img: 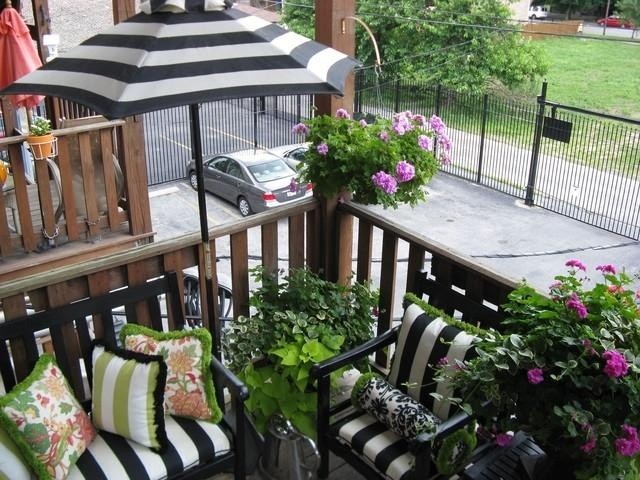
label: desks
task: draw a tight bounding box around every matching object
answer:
[457,429,543,480]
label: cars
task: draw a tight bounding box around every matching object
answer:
[185,143,312,217]
[597,16,631,29]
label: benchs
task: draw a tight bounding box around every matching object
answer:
[0,270,250,480]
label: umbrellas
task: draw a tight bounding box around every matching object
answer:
[0,0,370,358]
[1,0,52,135]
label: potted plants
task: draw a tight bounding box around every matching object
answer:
[27,116,54,158]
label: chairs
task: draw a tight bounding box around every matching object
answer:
[307,323,493,480]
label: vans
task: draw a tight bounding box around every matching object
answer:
[528,6,547,19]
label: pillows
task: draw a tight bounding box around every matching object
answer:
[388,294,504,433]
[0,352,98,480]
[0,424,35,479]
[119,321,223,425]
[358,378,443,446]
[89,336,170,454]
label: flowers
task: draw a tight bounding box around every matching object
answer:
[399,258,640,480]
[282,111,452,210]
